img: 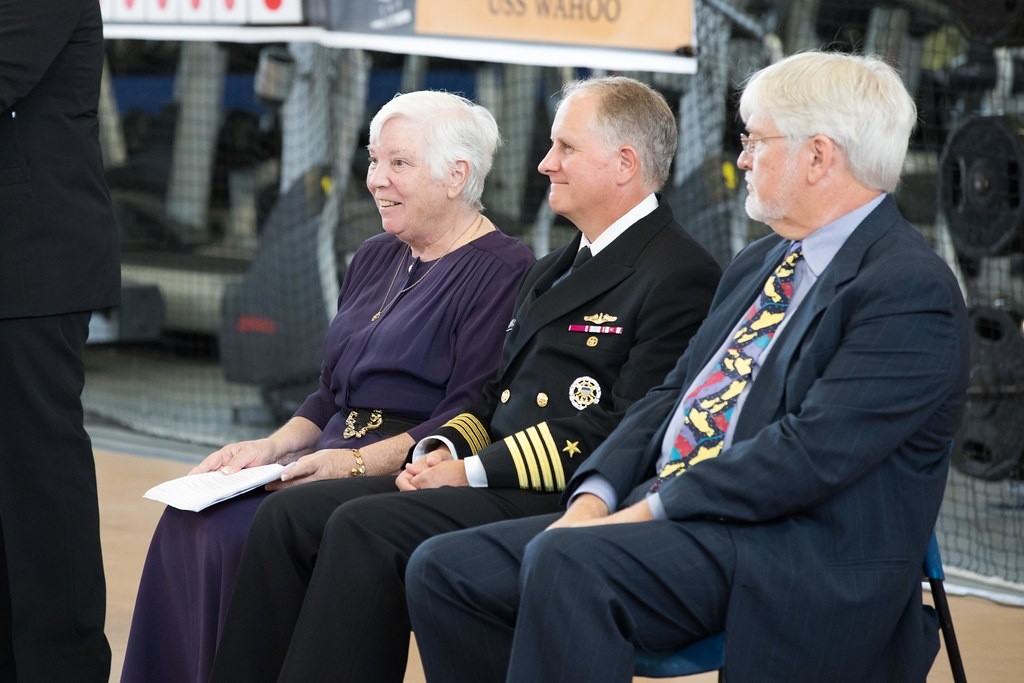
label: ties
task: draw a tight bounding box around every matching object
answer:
[571,245,592,274]
[644,245,804,493]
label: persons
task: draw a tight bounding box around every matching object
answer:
[0,0,122,683]
[119,90,537,683]
[405,49,971,683]
[205,77,724,683]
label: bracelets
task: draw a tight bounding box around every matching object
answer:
[347,449,366,478]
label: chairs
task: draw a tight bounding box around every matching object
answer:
[633,533,967,683]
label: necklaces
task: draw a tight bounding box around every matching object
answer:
[371,213,483,321]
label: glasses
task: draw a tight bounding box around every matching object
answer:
[739,133,788,149]
